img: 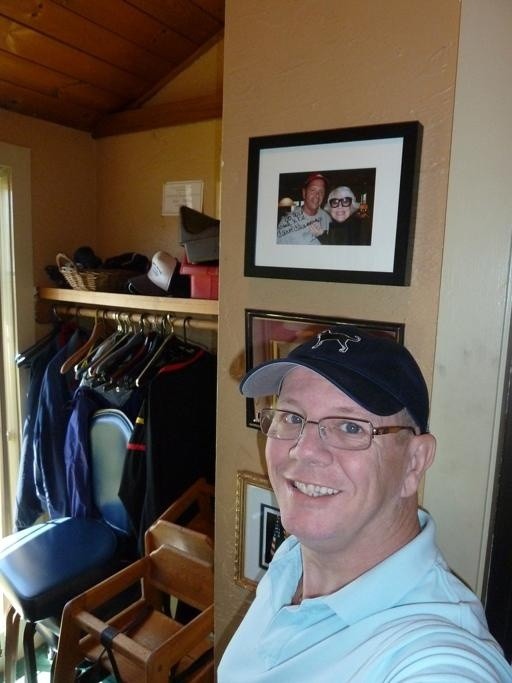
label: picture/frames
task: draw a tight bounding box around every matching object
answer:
[243,307,406,430]
[233,467,293,594]
[243,118,424,287]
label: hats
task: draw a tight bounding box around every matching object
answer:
[278,197,297,208]
[238,324,429,435]
[304,173,327,186]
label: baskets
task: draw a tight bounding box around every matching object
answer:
[50,251,139,293]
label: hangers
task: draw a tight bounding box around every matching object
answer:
[14,305,199,391]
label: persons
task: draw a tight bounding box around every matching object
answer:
[216,325,512,683]
[277,171,371,245]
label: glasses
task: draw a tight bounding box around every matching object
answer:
[329,197,352,208]
[253,409,419,451]
